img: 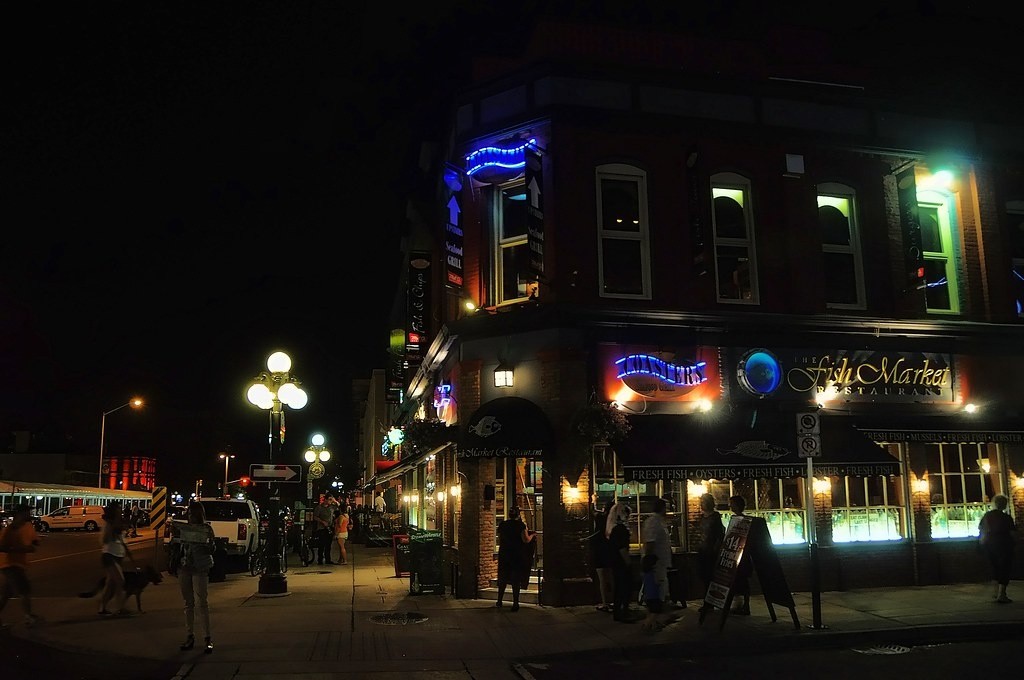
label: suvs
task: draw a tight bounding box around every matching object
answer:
[41,506,106,532]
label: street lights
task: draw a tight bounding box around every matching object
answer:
[303,431,332,548]
[220,454,236,497]
[245,352,308,597]
[99,401,143,489]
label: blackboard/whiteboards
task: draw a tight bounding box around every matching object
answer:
[409,531,446,595]
[393,534,410,572]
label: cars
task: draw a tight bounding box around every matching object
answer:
[0,509,13,528]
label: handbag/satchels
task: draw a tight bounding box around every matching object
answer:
[976,544,992,561]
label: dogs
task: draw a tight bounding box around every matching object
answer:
[80,570,167,615]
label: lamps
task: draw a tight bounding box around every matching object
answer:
[494,364,515,387]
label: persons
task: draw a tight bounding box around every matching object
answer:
[313,493,335,565]
[97,501,137,615]
[589,493,623,621]
[693,493,750,615]
[640,499,672,631]
[496,507,537,612]
[351,504,371,525]
[978,494,1016,604]
[375,491,386,516]
[179,502,216,653]
[124,506,137,536]
[0,504,44,624]
[933,494,943,504]
[334,504,349,565]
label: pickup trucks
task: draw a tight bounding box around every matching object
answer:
[163,498,259,572]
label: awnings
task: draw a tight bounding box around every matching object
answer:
[456,396,554,462]
[823,401,1024,445]
[591,401,902,485]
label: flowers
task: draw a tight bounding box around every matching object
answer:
[401,417,448,456]
[567,401,632,462]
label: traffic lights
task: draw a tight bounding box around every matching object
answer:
[242,479,247,483]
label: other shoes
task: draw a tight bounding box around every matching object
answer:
[992,592,1012,604]
[732,608,750,616]
[325,560,337,565]
[117,609,134,616]
[337,559,347,565]
[496,601,501,606]
[697,606,714,613]
[597,603,609,613]
[511,603,519,609]
[204,641,214,654]
[26,616,38,629]
[180,640,193,651]
[98,609,112,617]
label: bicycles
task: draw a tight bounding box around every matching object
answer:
[250,535,288,577]
[299,536,316,566]
